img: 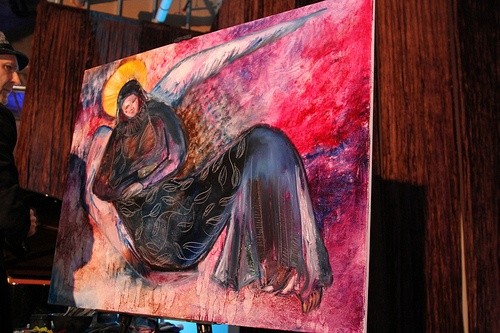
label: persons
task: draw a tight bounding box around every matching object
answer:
[0,30,37,306]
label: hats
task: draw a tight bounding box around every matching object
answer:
[0,30,29,71]
